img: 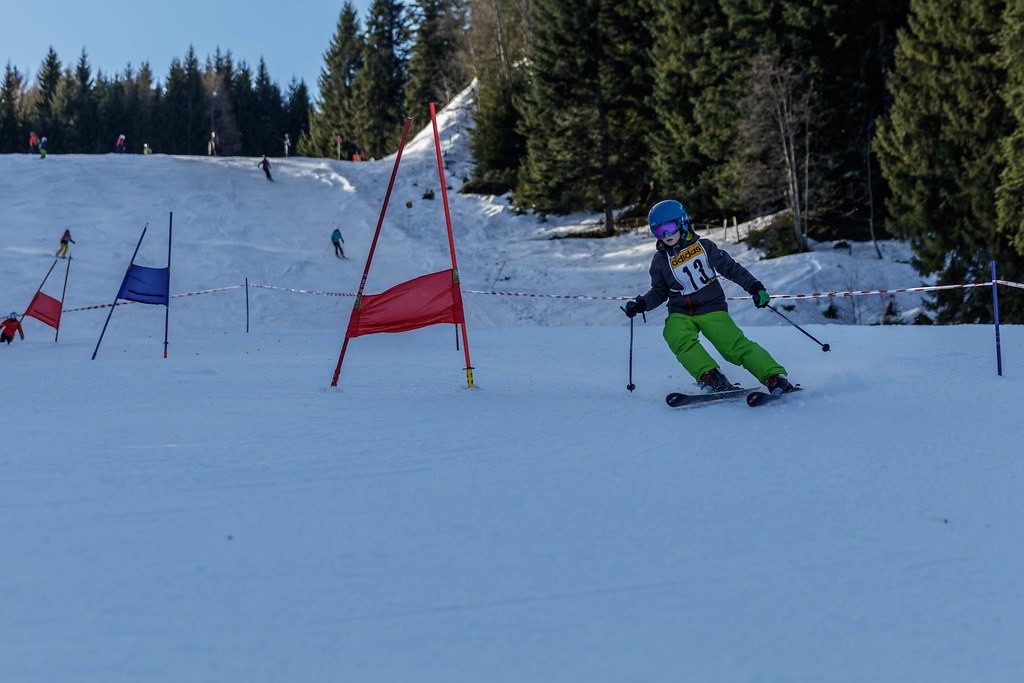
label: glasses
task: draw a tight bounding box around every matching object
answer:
[649,219,682,240]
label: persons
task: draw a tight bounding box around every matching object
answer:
[258,155,273,181]
[207,129,219,155]
[116,134,126,154]
[0,311,24,345]
[335,134,342,160]
[347,147,375,161]
[283,133,291,156]
[332,226,344,258]
[624,200,795,394]
[28,130,47,159]
[143,142,152,155]
[55,229,77,257]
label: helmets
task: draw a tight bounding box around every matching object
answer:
[648,199,688,240]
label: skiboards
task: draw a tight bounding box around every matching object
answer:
[664,382,803,408]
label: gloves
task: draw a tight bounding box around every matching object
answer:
[746,282,770,308]
[625,301,644,318]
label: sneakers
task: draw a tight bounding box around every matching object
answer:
[691,369,744,393]
[762,374,795,400]
